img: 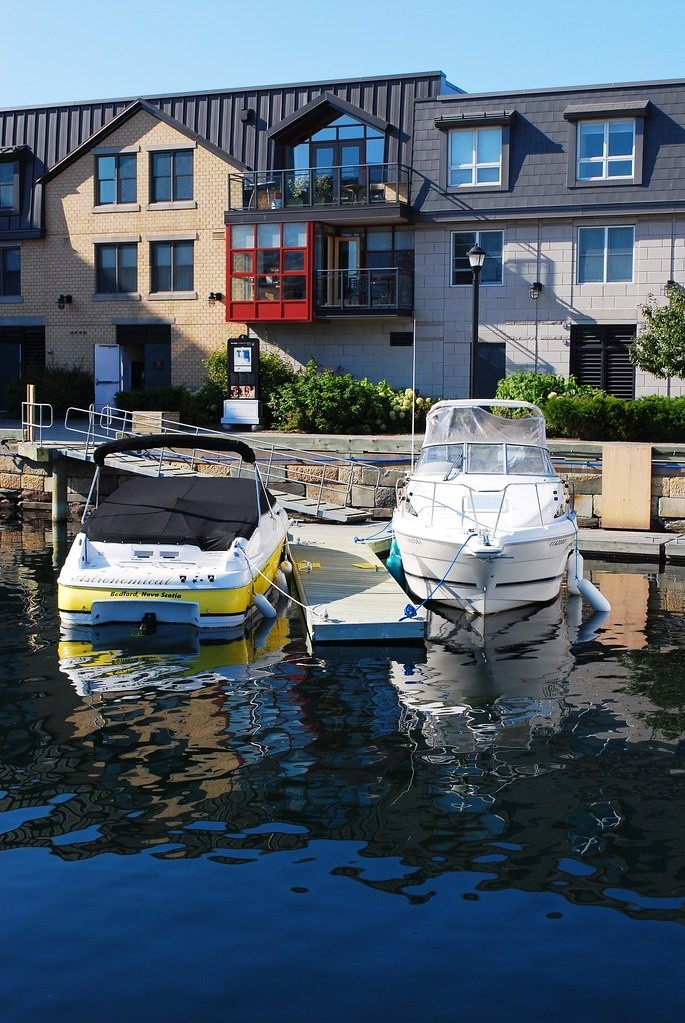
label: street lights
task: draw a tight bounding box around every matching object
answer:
[467,241,489,403]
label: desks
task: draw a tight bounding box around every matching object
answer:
[345,184,366,206]
[248,282,300,301]
[244,181,277,210]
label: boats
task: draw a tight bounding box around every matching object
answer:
[54,435,290,648]
[56,601,297,698]
[391,589,576,711]
[387,318,610,617]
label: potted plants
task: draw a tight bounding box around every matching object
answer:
[116,384,194,433]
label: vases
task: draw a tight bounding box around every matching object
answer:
[285,194,331,207]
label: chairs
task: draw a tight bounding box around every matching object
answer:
[349,275,395,305]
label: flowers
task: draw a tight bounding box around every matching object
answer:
[288,173,332,199]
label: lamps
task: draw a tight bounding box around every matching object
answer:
[55,294,73,311]
[209,292,222,308]
[530,282,542,299]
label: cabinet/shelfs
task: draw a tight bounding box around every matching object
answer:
[370,182,409,203]
